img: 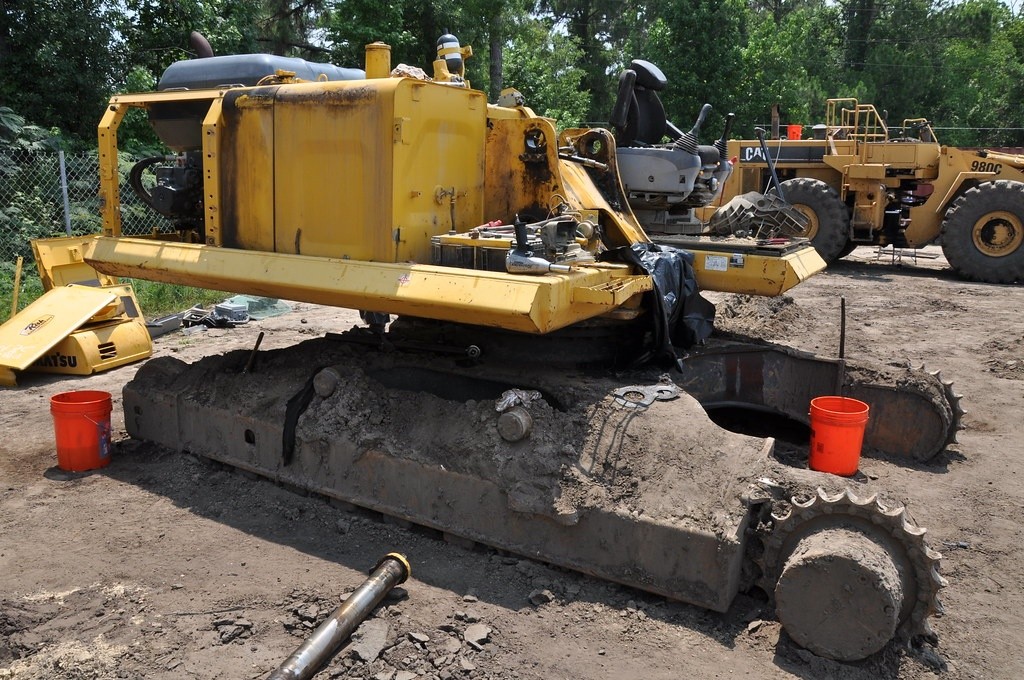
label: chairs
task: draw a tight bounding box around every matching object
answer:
[880,110,917,142]
[609,58,721,166]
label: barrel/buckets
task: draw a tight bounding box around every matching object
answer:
[50,390,113,471]
[808,396,869,476]
[787,125,802,140]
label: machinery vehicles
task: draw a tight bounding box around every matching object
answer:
[696,98,1024,285]
[86,38,970,680]
